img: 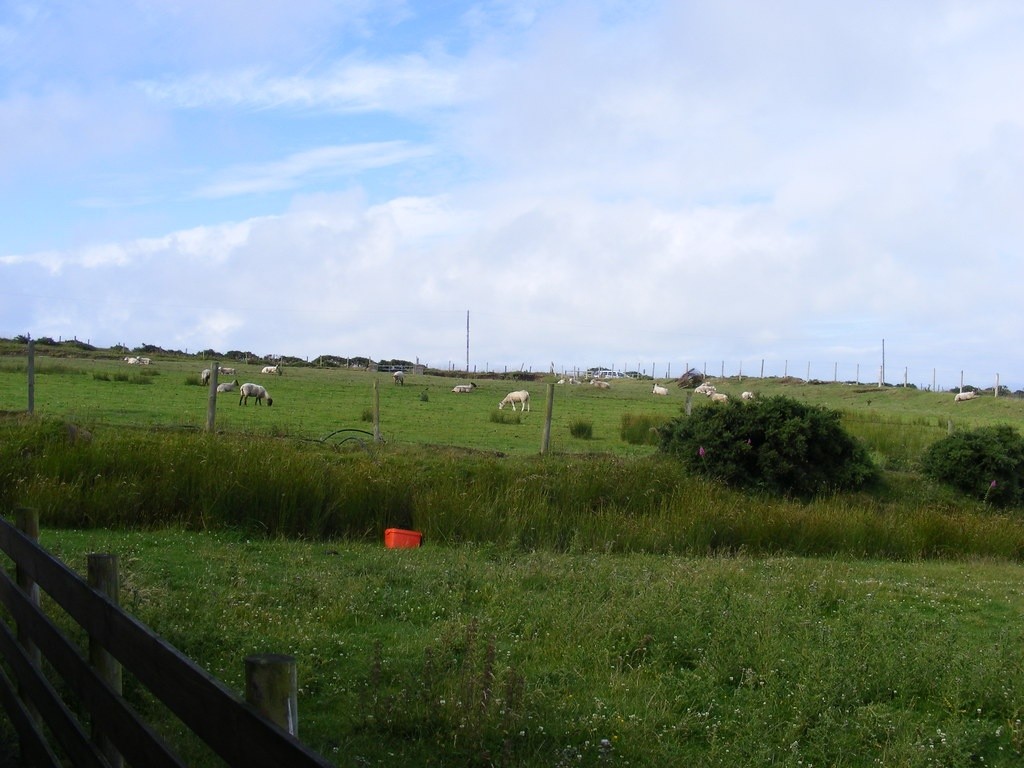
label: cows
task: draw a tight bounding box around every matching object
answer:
[742,391,756,399]
[499,390,531,412]
[124,356,153,366]
[558,378,581,385]
[392,371,404,385]
[239,383,273,406]
[261,365,278,373]
[652,382,668,396]
[216,379,239,392]
[710,390,728,403]
[590,379,609,389]
[202,368,211,386]
[218,367,236,375]
[451,382,477,393]
[955,390,976,401]
[694,381,716,396]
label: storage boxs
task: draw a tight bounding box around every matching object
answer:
[384,528,422,549]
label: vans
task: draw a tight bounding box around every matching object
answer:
[586,371,627,379]
